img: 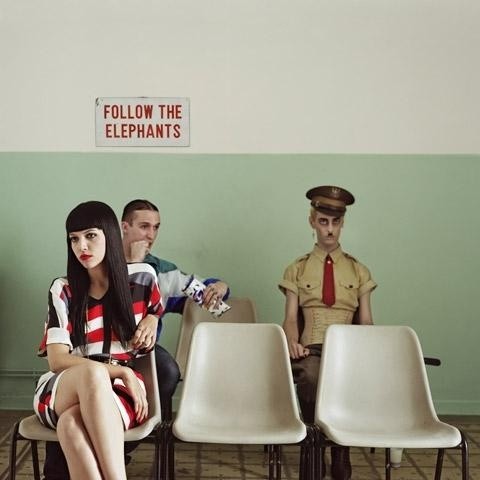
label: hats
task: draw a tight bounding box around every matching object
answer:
[306,187,355,217]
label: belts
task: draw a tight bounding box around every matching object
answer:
[83,355,134,369]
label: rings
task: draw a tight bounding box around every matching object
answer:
[213,295,218,301]
[138,327,144,334]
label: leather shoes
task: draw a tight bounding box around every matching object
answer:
[330,446,351,480]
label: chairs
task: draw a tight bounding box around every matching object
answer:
[167,322,313,480]
[170,296,259,381]
[9,343,166,479]
[314,322,467,478]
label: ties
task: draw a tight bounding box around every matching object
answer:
[320,253,335,308]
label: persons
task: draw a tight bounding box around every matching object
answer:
[44,200,229,479]
[33,200,165,479]
[277,186,379,478]
